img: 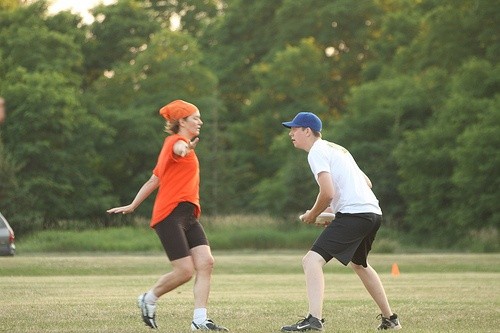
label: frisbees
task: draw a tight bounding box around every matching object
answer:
[300,211,335,225]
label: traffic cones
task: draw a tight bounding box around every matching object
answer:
[392,262,400,276]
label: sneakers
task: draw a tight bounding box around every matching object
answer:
[376,313,402,331]
[136,292,158,329]
[192,319,228,332]
[280,313,325,331]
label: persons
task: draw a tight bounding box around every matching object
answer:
[106,99,229,332]
[280,112,402,331]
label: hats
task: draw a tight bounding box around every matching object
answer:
[282,112,322,133]
[159,99,198,122]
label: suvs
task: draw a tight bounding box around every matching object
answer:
[1,213,17,257]
